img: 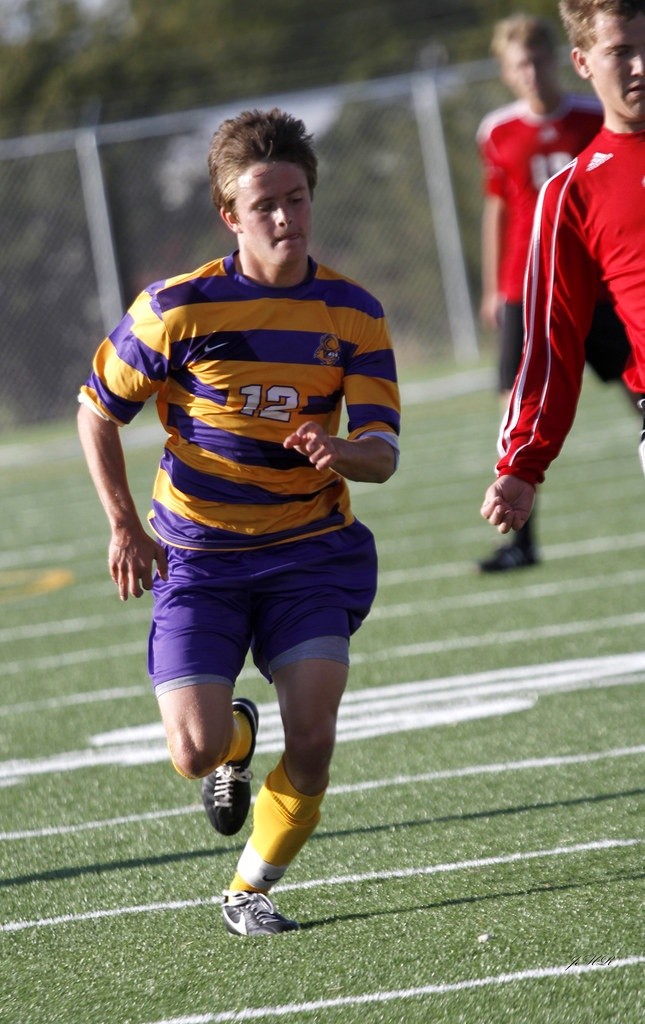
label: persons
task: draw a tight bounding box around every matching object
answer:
[77,107,402,936]
[479,0,645,535]
[469,17,641,573]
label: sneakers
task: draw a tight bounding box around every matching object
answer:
[220,889,301,939]
[200,699,259,836]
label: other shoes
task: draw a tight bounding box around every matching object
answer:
[479,545,540,571]
